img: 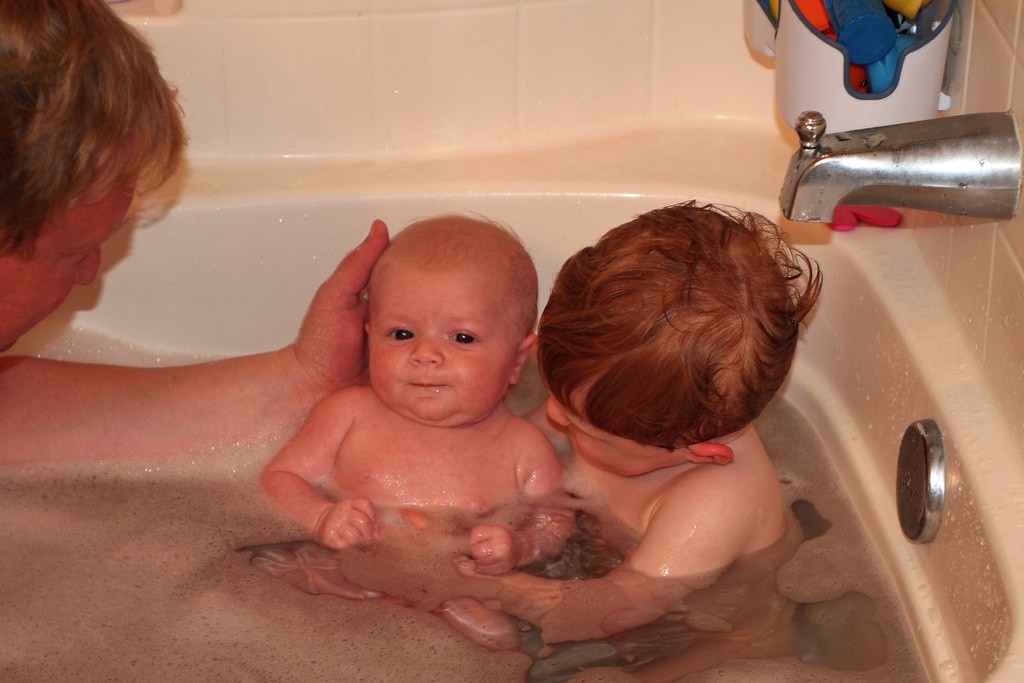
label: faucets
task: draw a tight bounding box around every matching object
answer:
[777,108,1024,224]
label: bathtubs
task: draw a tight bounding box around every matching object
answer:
[0,191,1024,682]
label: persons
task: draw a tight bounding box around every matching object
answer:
[0,0,390,481]
[256,200,823,640]
